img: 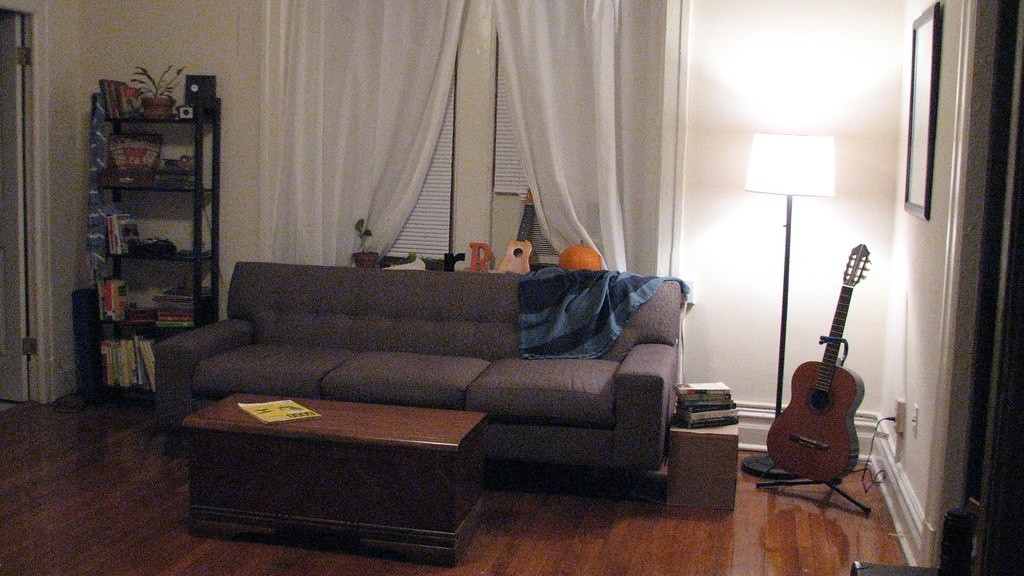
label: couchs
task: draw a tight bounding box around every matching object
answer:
[153,263,681,500]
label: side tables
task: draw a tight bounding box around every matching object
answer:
[666,415,738,511]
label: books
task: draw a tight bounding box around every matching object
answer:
[669,381,738,429]
[237,399,324,427]
[97,80,196,393]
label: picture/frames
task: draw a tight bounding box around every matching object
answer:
[903,0,942,220]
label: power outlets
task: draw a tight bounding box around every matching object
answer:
[911,404,919,439]
[893,398,906,433]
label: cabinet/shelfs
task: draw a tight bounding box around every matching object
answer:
[89,92,221,402]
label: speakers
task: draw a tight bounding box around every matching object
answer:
[185,74,216,122]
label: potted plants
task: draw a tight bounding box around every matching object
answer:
[352,218,379,267]
[130,65,187,119]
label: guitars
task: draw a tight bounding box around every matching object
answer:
[766,244,872,481]
[495,188,534,275]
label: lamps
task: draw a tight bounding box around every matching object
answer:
[742,133,836,480]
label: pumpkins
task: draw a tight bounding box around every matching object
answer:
[559,239,601,270]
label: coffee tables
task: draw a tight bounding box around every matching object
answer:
[182,393,492,568]
